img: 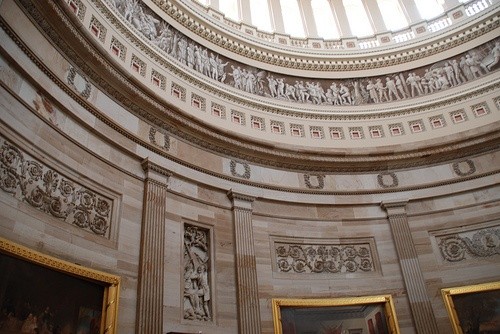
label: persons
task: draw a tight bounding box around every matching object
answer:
[183,263,211,319]
[109,0,500,106]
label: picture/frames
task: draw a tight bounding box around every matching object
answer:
[441,281,500,334]
[271,293,400,334]
[0,239,120,334]
